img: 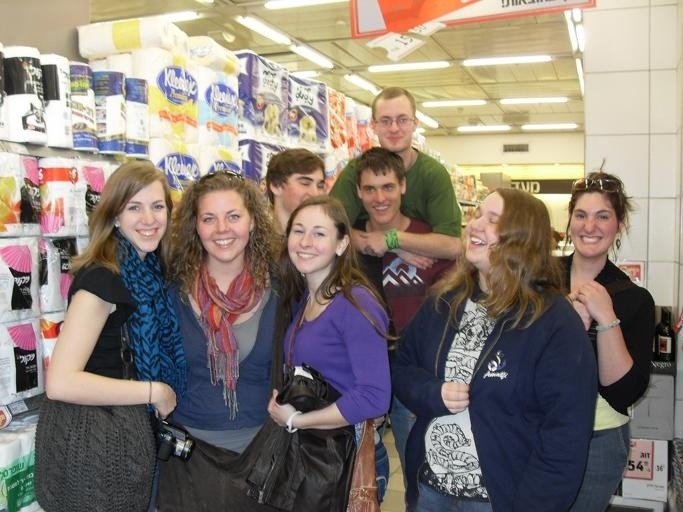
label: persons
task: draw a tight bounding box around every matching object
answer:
[33,157,188,511]
[386,184,598,512]
[265,147,326,235]
[349,145,458,339]
[328,86,463,271]
[261,191,399,511]
[548,170,658,511]
[157,168,288,512]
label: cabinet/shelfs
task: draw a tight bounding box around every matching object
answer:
[0,138,142,426]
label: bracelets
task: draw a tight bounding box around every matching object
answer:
[384,227,400,250]
[284,411,301,434]
[147,379,153,406]
[594,319,622,330]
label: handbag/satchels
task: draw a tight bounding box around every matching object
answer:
[347,413,381,512]
[35,378,156,512]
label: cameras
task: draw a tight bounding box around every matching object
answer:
[156,421,195,463]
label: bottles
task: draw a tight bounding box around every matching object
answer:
[652,307,674,364]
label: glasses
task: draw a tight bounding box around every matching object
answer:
[372,116,414,127]
[199,169,243,185]
[572,178,620,194]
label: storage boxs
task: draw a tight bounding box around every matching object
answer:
[627,371,675,442]
[620,437,669,504]
